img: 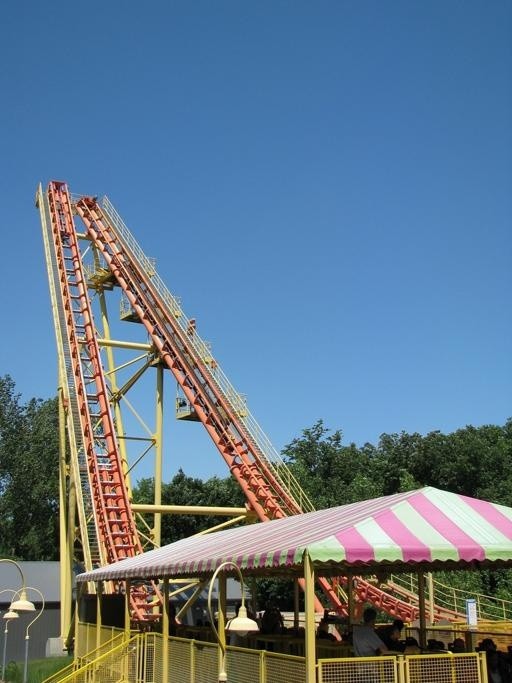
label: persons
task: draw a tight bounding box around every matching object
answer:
[228,604,252,648]
[376,622,408,654]
[403,638,512,682]
[257,599,285,652]
[350,608,387,656]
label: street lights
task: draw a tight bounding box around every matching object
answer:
[207,561,260,683]
[0,559,47,682]
